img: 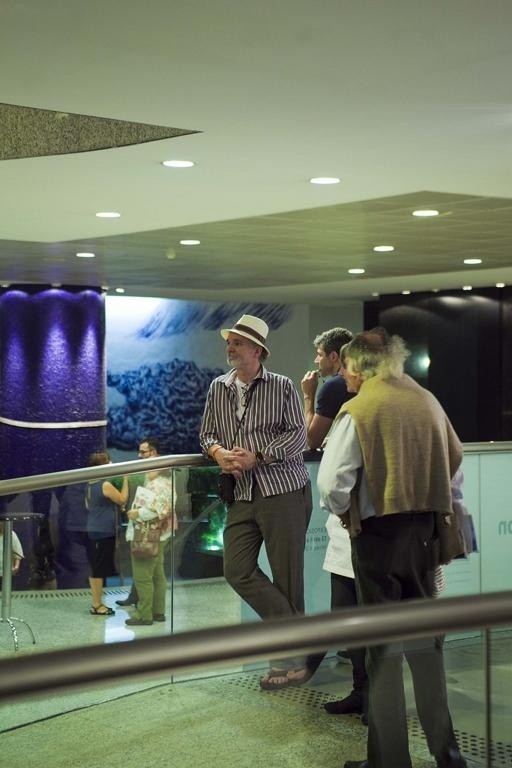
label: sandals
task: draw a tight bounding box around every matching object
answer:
[89,604,116,614]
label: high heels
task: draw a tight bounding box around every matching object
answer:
[115,597,137,608]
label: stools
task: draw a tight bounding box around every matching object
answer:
[0,511,45,652]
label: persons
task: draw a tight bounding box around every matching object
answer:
[0,520,24,592]
[83,438,179,627]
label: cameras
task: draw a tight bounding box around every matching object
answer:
[217,472,236,506]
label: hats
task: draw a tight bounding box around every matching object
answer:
[213,315,274,355]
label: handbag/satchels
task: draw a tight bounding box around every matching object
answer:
[218,471,235,504]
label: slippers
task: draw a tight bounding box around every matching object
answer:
[258,646,327,690]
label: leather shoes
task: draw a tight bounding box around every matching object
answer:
[325,692,369,727]
[124,613,165,626]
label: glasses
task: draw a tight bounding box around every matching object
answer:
[136,448,154,454]
